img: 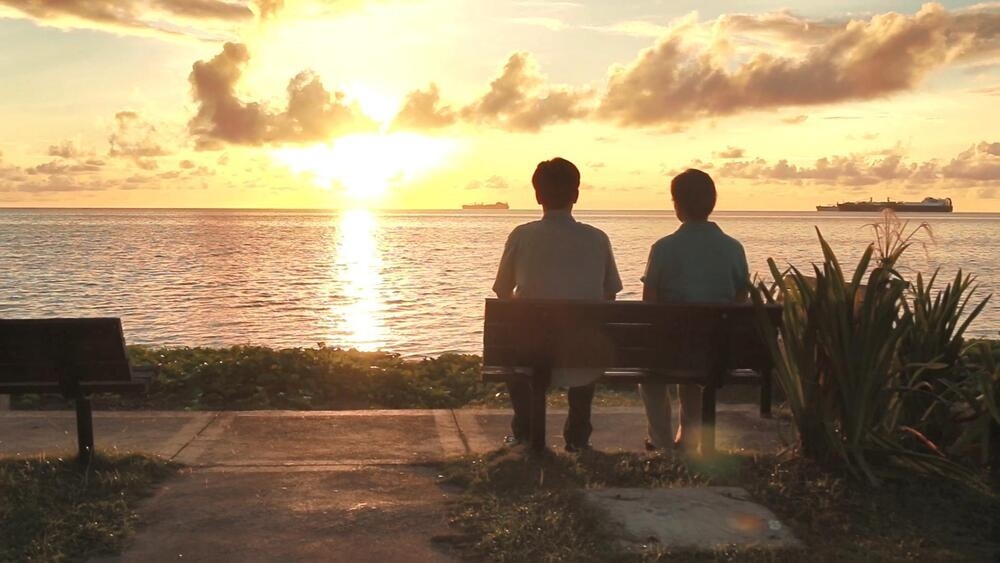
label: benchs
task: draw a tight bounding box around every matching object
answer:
[0,316,156,457]
[480,297,781,457]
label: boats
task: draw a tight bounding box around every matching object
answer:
[462,201,510,210]
[817,203,840,212]
[835,197,954,214]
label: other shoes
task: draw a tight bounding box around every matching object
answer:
[503,435,515,444]
[645,437,659,452]
[565,438,593,452]
[674,440,699,452]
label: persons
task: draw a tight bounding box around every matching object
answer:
[640,169,751,449]
[492,157,623,453]
